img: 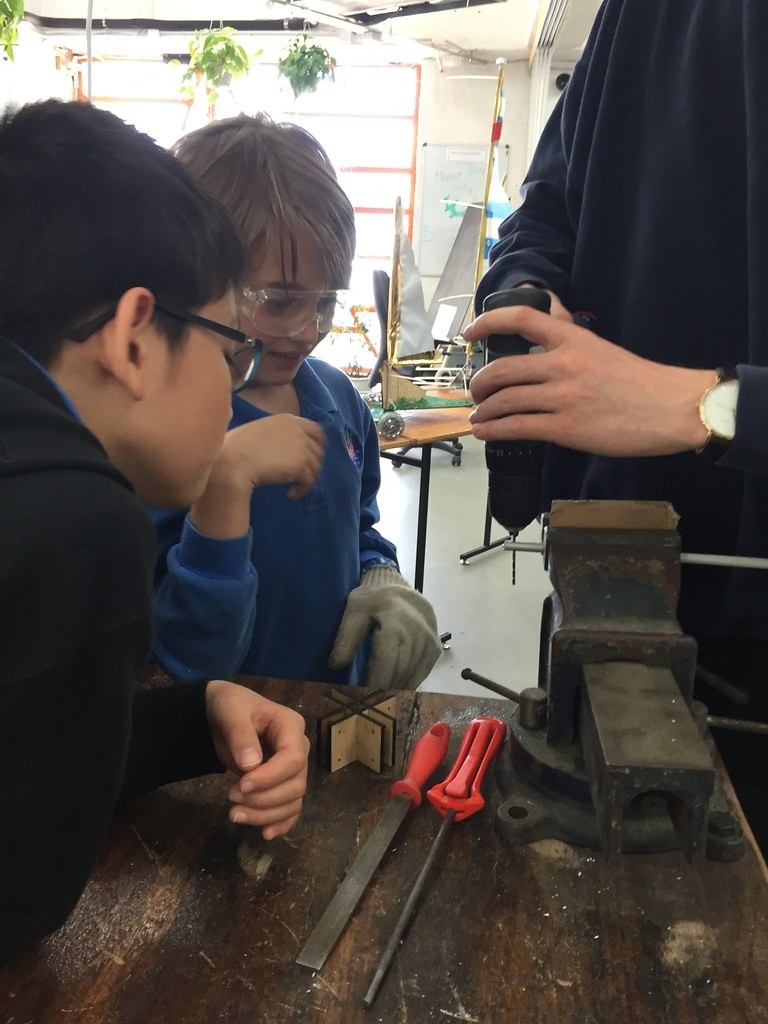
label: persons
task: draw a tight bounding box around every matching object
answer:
[462,0,768,843]
[140,117,442,709]
[0,103,307,954]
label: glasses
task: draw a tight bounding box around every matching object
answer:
[231,282,354,338]
[65,299,262,395]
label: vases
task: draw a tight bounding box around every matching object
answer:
[290,77,319,93]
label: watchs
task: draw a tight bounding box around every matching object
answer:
[695,366,738,461]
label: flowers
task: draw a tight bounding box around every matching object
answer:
[275,33,338,101]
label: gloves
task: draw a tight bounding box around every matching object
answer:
[329,566,443,691]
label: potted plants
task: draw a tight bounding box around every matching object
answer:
[346,356,371,394]
[168,26,249,107]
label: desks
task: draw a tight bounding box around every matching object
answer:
[362,384,512,596]
[0,691,768,1024]
